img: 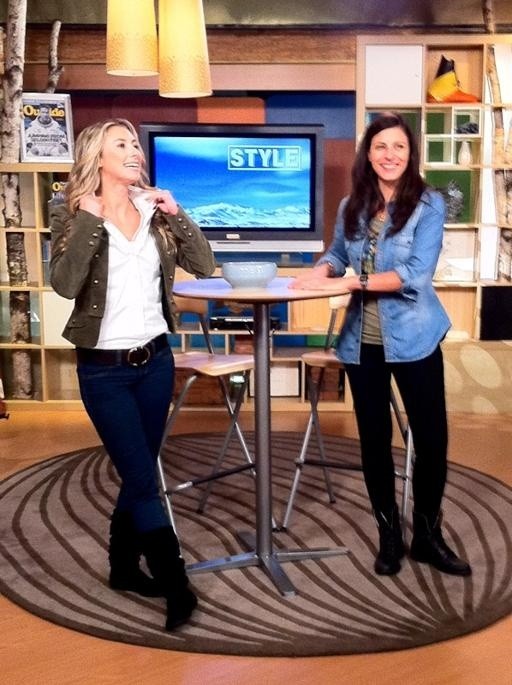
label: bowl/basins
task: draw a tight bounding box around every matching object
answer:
[221,260,279,289]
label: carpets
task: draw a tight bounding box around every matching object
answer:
[1,427,511,658]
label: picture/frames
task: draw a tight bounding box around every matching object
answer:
[20,91,76,164]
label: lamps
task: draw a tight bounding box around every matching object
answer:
[105,0,158,78]
[159,0,213,98]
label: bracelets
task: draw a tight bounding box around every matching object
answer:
[327,262,334,277]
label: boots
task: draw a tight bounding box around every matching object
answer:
[410,507,472,576]
[109,509,198,631]
[373,506,404,574]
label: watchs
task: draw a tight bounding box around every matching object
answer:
[360,272,368,291]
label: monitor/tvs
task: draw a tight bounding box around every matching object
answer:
[138,121,325,252]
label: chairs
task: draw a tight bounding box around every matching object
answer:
[282,265,414,543]
[157,267,280,560]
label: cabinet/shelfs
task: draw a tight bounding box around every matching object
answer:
[165,266,353,404]
[355,32,512,414]
[0,163,95,426]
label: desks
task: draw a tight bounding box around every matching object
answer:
[172,276,355,597]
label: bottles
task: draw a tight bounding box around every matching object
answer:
[457,140,471,166]
[228,374,245,405]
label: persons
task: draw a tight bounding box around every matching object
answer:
[25,103,68,156]
[48,118,215,632]
[288,111,472,578]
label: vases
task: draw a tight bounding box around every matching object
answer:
[457,141,473,166]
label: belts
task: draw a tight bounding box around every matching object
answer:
[75,333,169,367]
